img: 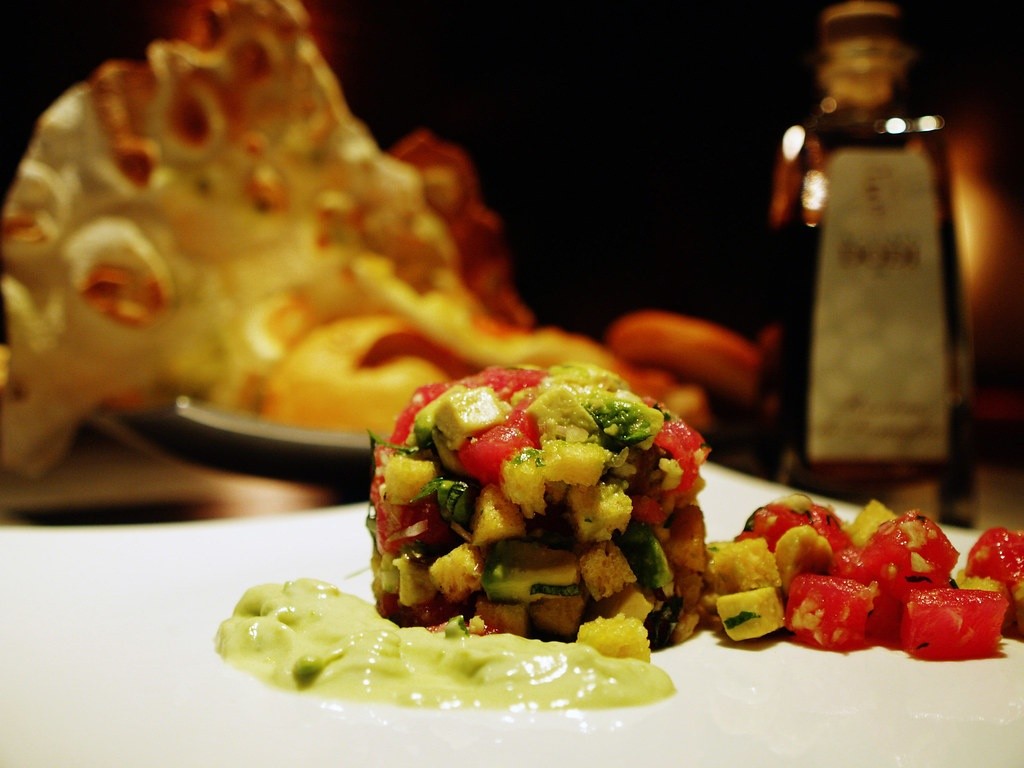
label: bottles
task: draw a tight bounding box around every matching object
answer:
[773,0,980,530]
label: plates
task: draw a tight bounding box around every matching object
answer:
[0,453,1024,768]
[168,390,415,479]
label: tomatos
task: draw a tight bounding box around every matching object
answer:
[734,494,1024,660]
[365,365,701,554]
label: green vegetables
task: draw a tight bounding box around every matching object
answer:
[365,397,764,649]
